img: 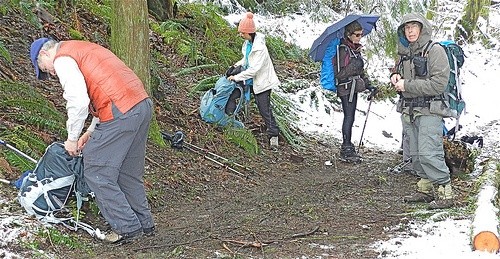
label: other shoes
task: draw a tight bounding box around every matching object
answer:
[142,226,156,236]
[270,136,278,150]
[104,226,143,244]
[404,191,435,203]
[430,196,453,209]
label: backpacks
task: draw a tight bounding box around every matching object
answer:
[422,41,465,118]
[200,76,244,128]
[18,141,91,220]
[320,37,354,91]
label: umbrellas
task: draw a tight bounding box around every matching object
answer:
[308,13,380,59]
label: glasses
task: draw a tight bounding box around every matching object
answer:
[402,23,420,32]
[351,33,364,37]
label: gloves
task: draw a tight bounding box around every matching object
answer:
[368,86,377,92]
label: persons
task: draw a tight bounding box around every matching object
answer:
[226,12,282,150]
[29,38,156,248]
[334,20,373,164]
[388,13,455,210]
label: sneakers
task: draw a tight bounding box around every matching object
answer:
[339,143,360,163]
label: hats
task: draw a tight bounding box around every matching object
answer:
[238,12,256,33]
[30,38,48,80]
